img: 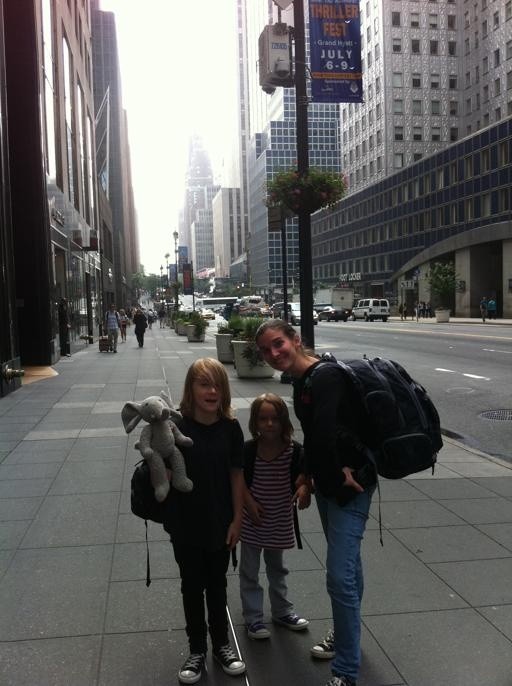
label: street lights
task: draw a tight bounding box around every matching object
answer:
[159,229,180,314]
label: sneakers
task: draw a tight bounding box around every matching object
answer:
[246,621,271,638]
[325,675,356,686]
[178,653,204,683]
[310,629,336,658]
[273,613,309,630]
[212,644,246,675]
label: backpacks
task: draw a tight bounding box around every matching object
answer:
[304,350,443,480]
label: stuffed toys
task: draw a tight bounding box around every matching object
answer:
[120,388,196,501]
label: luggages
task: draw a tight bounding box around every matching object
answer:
[99,329,109,352]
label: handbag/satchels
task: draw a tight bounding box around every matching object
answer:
[130,459,163,524]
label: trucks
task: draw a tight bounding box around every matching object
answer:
[313,286,355,314]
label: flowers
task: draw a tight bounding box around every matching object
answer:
[263,158,349,215]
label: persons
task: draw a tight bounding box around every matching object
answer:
[479,295,497,322]
[254,316,382,686]
[236,391,315,644]
[396,300,433,321]
[103,302,168,353]
[162,357,247,685]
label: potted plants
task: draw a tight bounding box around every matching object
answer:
[424,261,462,323]
[171,309,275,379]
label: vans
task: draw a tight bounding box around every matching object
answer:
[349,296,391,322]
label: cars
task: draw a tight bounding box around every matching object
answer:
[181,289,349,326]
